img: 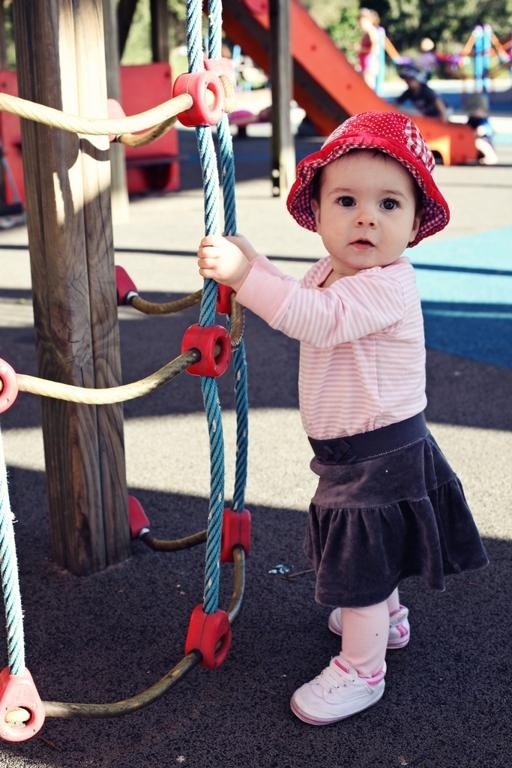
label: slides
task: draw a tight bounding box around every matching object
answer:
[197,1,477,167]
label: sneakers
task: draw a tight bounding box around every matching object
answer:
[327,605,413,651]
[289,655,389,727]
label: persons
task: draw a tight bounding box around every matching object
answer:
[463,91,500,166]
[196,108,493,726]
[352,6,386,94]
[393,60,452,122]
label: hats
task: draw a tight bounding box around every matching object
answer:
[286,110,452,251]
